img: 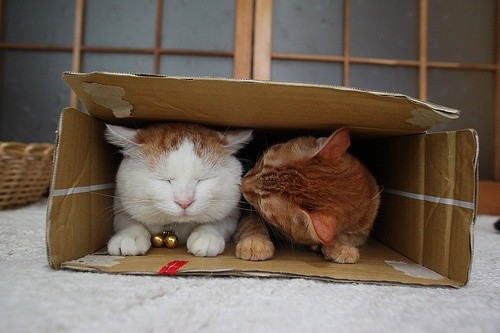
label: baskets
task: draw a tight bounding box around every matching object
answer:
[1,141,56,210]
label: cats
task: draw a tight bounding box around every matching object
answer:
[236,127,383,264]
[102,121,255,258]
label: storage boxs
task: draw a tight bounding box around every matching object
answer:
[44,71,480,288]
